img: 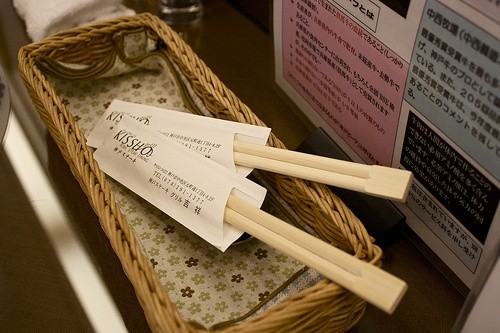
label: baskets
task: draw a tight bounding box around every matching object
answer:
[17,13,383,333]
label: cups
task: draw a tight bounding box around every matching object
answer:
[160,0,203,25]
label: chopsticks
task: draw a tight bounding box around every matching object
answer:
[232,140,414,202]
[223,189,408,314]
